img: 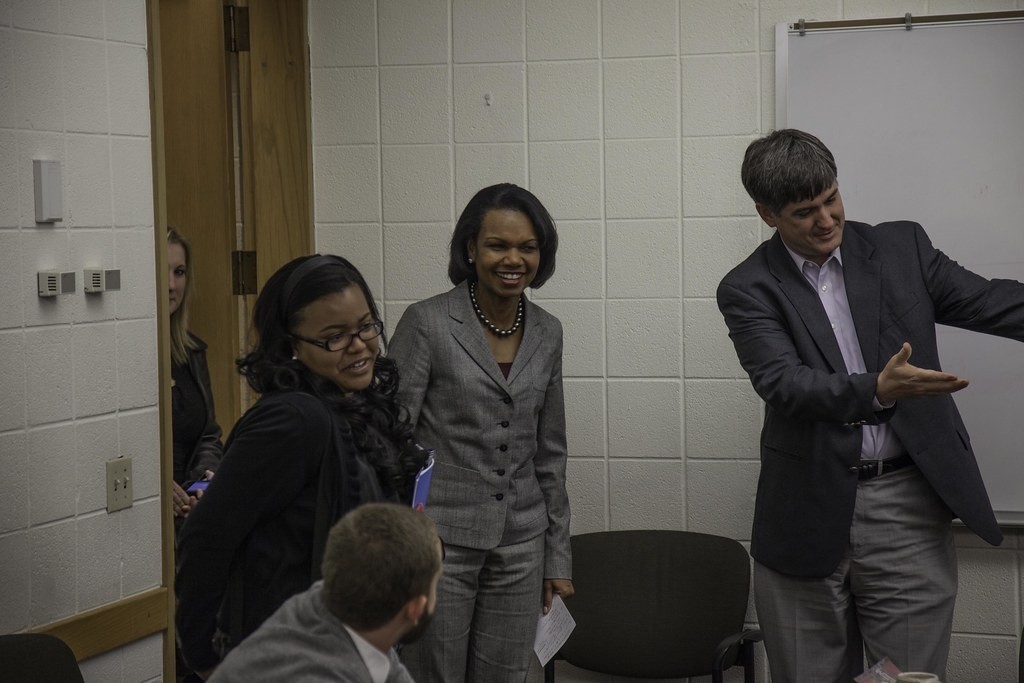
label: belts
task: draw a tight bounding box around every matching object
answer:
[858,454,915,480]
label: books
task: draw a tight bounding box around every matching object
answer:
[412,441,435,513]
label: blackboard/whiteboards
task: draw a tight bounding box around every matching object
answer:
[771,9,1023,553]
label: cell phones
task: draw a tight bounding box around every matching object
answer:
[186,481,209,497]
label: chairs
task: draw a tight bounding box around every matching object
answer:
[544,530,763,683]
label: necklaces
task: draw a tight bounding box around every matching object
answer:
[470,279,525,338]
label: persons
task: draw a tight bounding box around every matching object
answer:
[715,127,1023,683]
[171,250,436,682]
[164,222,226,648]
[384,181,578,683]
[199,502,444,683]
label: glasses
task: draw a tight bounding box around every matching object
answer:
[288,315,383,352]
